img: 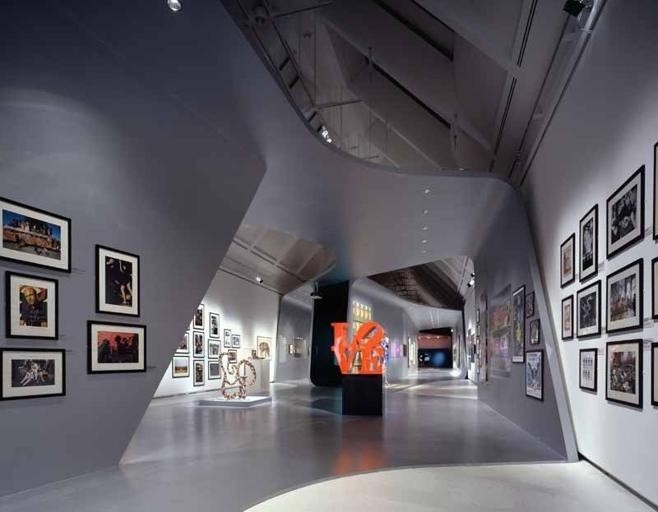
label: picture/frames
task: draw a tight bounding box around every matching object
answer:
[0,348,67,401]
[0,196,72,272]
[172,303,241,387]
[559,141,657,409]
[466,284,544,402]
[87,319,147,374]
[94,243,140,317]
[4,270,58,340]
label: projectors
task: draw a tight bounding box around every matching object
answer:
[311,281,323,299]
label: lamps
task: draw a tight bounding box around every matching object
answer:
[165,0,179,12]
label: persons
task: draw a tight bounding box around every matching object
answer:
[20,286,47,327]
[20,360,48,387]
[99,334,138,362]
[110,259,132,303]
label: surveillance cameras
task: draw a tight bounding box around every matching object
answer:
[467,280,476,288]
[256,273,264,284]
